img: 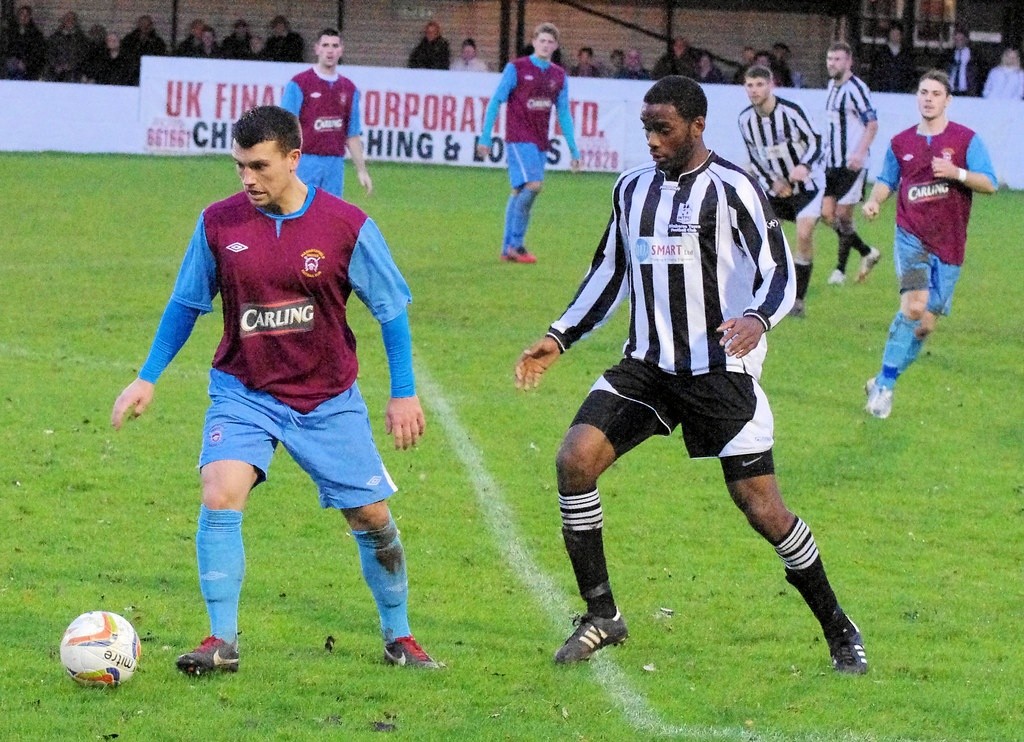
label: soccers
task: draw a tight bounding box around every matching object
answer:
[59,610,142,688]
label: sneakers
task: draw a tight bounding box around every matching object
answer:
[828,270,849,286]
[855,247,880,282]
[555,605,628,664]
[828,615,867,675]
[176,635,239,674]
[384,634,440,670]
[501,246,536,263]
[864,378,893,420]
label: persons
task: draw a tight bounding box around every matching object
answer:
[279,28,373,200]
[0,0,304,87]
[739,66,827,318]
[868,20,1024,102]
[111,105,441,669]
[861,68,998,418]
[572,36,808,88]
[407,23,488,74]
[477,23,583,262]
[820,42,881,284]
[509,76,867,673]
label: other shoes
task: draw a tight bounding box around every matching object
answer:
[789,298,804,315]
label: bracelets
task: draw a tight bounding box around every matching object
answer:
[957,168,967,182]
